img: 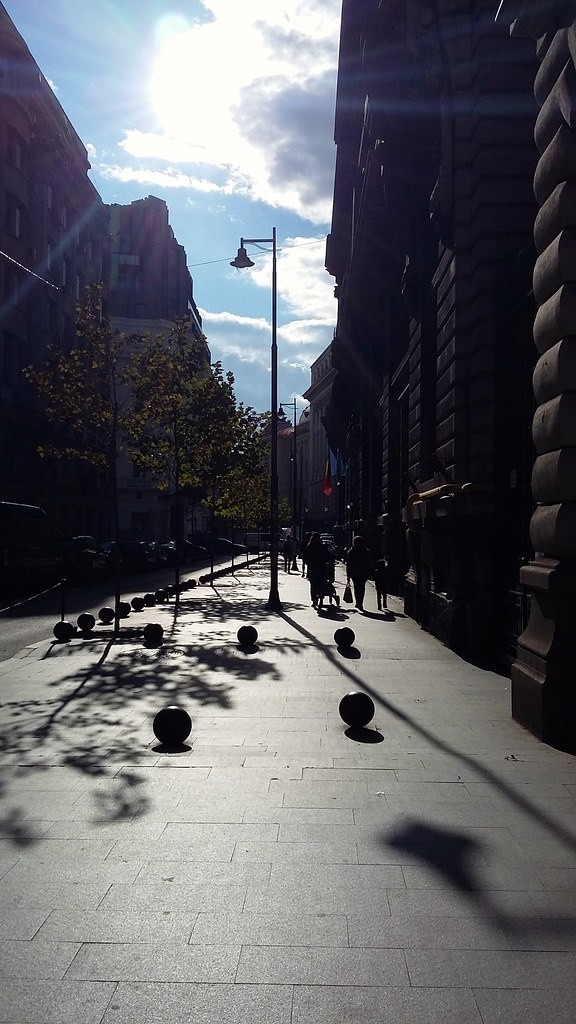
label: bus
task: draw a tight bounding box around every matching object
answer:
[1,501,58,598]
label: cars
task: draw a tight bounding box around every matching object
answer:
[321,533,348,561]
[59,533,248,582]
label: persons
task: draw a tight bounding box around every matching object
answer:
[374,559,392,611]
[305,532,333,611]
[346,535,374,611]
[279,531,349,579]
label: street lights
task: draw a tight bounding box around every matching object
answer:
[230,227,283,612]
[288,444,303,541]
[278,398,298,570]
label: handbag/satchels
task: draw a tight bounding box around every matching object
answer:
[343,582,354,603]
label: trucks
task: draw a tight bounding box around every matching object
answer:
[242,533,285,555]
